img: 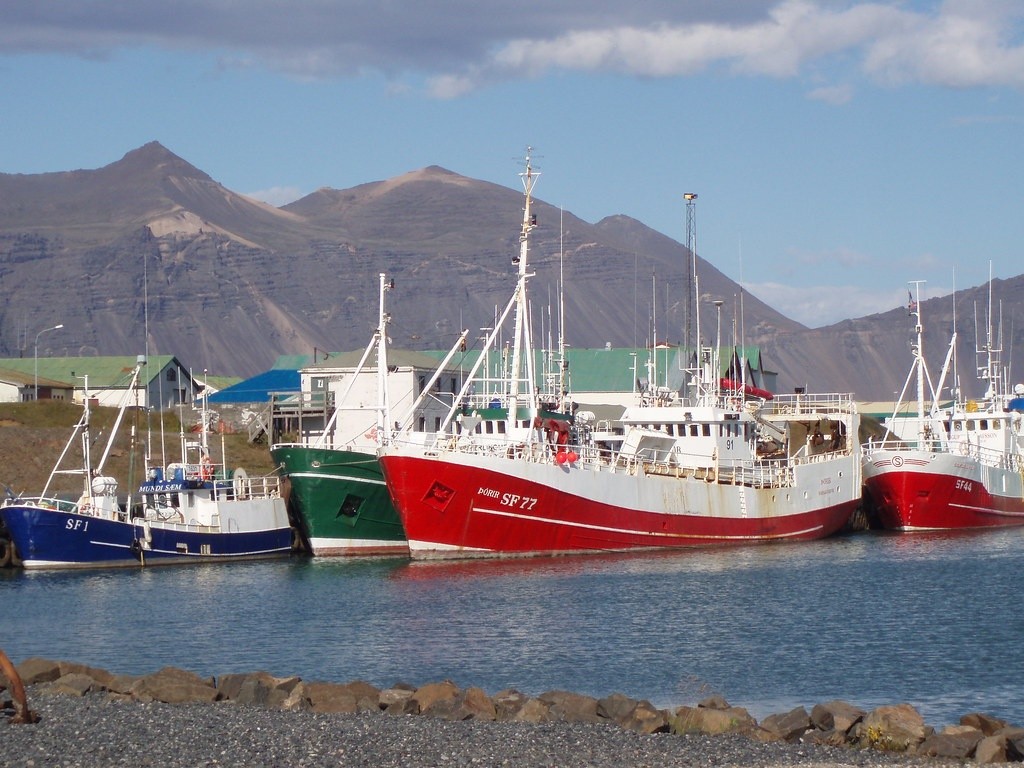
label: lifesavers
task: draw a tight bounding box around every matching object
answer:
[202,457,214,475]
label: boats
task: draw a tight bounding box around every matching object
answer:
[861,259,1024,535]
[380,145,864,556]
[0,254,293,568]
[267,200,621,553]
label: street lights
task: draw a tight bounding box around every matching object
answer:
[35,325,63,399]
